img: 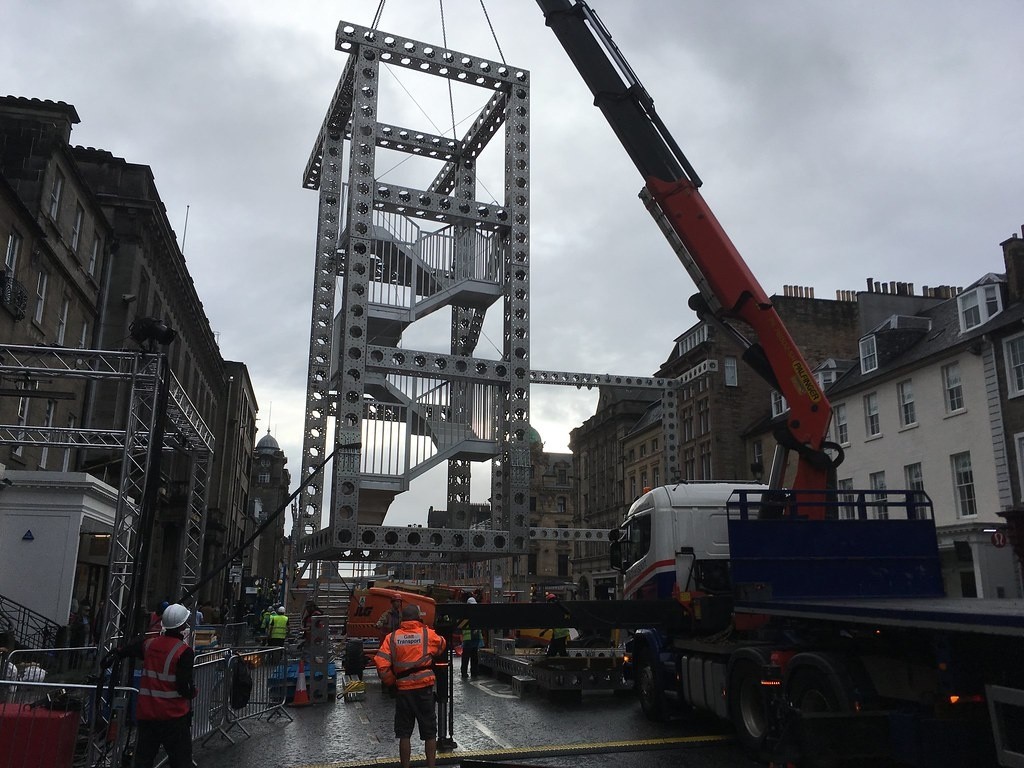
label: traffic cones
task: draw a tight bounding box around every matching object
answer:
[288,660,311,706]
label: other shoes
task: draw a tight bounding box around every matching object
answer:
[462,673,469,679]
[471,673,480,679]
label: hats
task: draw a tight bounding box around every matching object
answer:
[402,604,420,621]
[277,606,286,614]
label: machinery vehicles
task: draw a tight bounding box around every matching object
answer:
[343,0,1024,768]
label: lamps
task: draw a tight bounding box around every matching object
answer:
[110,236,120,255]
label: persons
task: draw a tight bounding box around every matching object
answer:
[121,603,198,768]
[374,604,446,768]
[196,606,203,624]
[220,597,230,621]
[377,594,402,699]
[236,603,255,641]
[259,606,278,636]
[199,601,217,623]
[70,598,161,653]
[298,600,327,648]
[270,606,290,658]
[461,597,482,680]
[539,593,570,657]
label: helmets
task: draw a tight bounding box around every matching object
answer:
[467,596,477,603]
[162,601,169,609]
[544,594,558,601]
[160,603,191,629]
[389,594,404,603]
[267,606,274,611]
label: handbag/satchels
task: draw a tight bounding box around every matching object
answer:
[230,653,252,711]
